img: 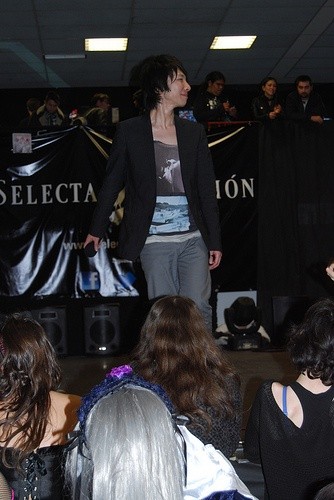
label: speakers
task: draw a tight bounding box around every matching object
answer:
[82,305,120,355]
[29,307,67,354]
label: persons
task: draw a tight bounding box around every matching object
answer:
[252,76,286,121]
[14,97,42,130]
[61,364,259,500]
[131,296,244,460]
[286,75,326,126]
[132,89,146,118]
[0,311,85,500]
[83,55,222,339]
[244,263,334,500]
[192,71,240,129]
[81,93,111,129]
[31,91,67,130]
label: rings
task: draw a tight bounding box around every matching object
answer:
[210,255,214,257]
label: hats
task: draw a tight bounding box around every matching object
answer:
[224,297,262,334]
